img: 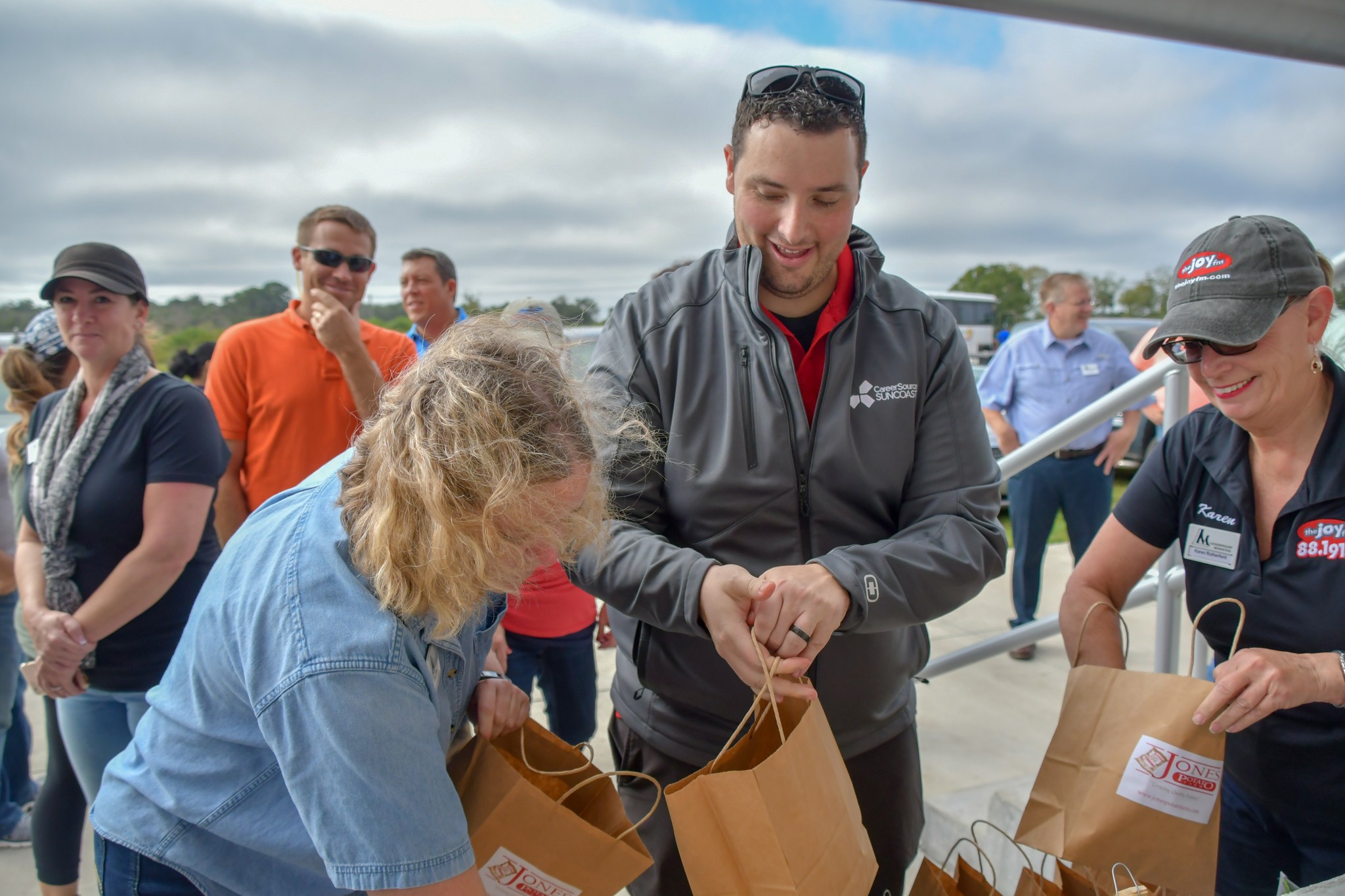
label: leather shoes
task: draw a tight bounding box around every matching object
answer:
[1009,643,1035,659]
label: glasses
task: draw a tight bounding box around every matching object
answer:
[1160,296,1302,363]
[741,66,865,116]
[298,244,373,273]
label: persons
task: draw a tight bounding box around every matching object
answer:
[15,242,232,803]
[489,298,617,746]
[206,205,417,551]
[0,306,86,894]
[92,312,673,896]
[1314,248,1345,372]
[400,248,469,357]
[974,272,1155,660]
[170,343,217,392]
[1061,215,1345,896]
[565,66,1009,896]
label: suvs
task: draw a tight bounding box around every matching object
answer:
[1010,316,1169,476]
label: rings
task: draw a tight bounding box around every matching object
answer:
[789,624,811,643]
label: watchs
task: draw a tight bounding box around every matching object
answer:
[1332,651,1344,709]
[479,671,510,682]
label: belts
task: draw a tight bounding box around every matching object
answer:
[1046,441,1107,460]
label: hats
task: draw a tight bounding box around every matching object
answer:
[1142,213,1326,361]
[40,242,149,301]
[22,307,67,366]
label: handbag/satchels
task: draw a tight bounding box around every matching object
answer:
[1011,596,1246,895]
[664,625,879,896]
[910,819,1168,896]
[445,715,661,894]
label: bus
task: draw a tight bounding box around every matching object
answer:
[920,292,998,368]
